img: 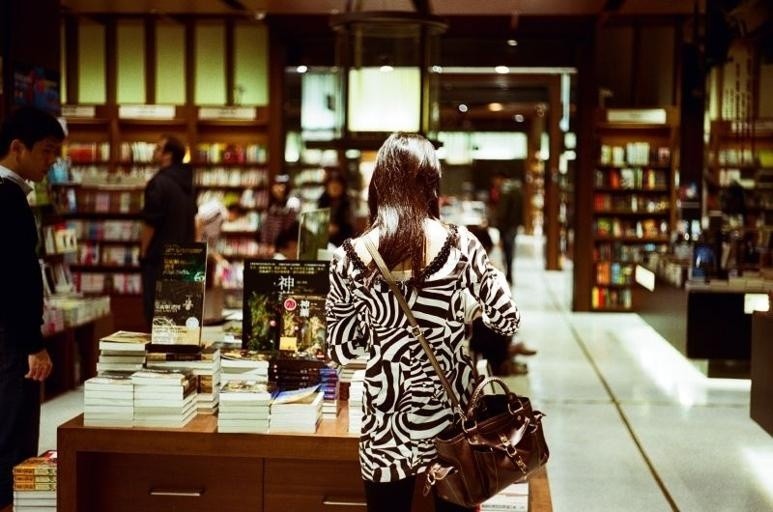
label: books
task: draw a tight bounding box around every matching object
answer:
[152,242,208,346]
[83,308,364,439]
[12,449,58,512]
[242,257,330,363]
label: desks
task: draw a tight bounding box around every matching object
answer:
[631,259,770,379]
[53,387,376,512]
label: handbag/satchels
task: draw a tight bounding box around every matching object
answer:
[363,231,550,507]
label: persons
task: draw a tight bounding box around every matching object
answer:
[490,166,523,291]
[273,227,318,259]
[325,132,520,512]
[317,173,355,244]
[194,198,245,327]
[0,107,65,430]
[262,175,300,256]
[138,135,197,334]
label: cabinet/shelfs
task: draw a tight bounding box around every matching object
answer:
[2,175,101,416]
[569,126,680,314]
[44,106,279,331]
